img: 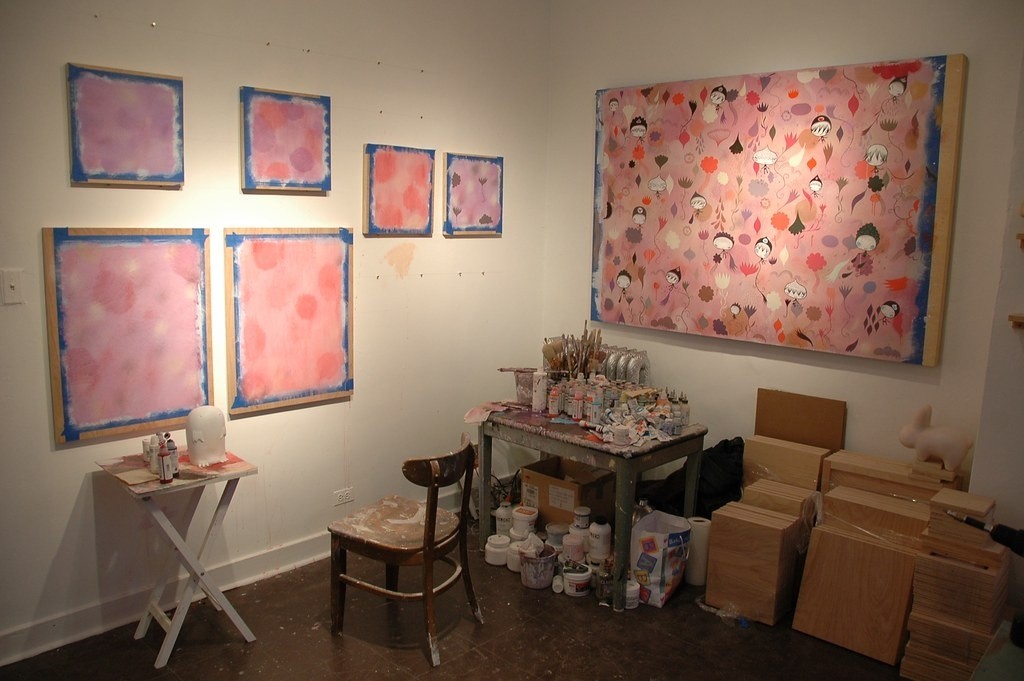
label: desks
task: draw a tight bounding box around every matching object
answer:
[476,407,710,612]
[95,443,260,668]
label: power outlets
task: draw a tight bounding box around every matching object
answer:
[344,486,356,503]
[333,489,346,506]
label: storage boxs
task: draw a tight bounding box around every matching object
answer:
[518,457,617,531]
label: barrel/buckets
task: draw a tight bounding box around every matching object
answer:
[513,507,538,532]
[570,523,589,553]
[558,553,585,575]
[545,540,562,556]
[510,527,528,542]
[520,546,556,590]
[563,534,583,561]
[563,564,592,597]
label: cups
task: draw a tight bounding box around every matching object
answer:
[532,372,547,413]
[546,522,569,545]
[574,506,591,529]
[516,367,535,404]
[553,574,565,593]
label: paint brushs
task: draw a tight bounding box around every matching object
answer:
[545,320,607,381]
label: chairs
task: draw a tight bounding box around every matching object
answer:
[325,432,485,667]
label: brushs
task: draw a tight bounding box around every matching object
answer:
[496,368,538,372]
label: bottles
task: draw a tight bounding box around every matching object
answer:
[554,565,558,576]
[485,534,509,565]
[631,499,653,526]
[598,566,614,603]
[150,435,162,473]
[588,516,612,559]
[158,446,174,485]
[589,561,600,585]
[495,502,512,534]
[507,542,525,571]
[547,371,689,433]
[164,439,179,479]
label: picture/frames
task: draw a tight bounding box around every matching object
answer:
[41,226,216,443]
[222,227,356,415]
[443,153,504,236]
[65,62,186,186]
[240,84,332,192]
[361,142,437,236]
[589,53,970,367]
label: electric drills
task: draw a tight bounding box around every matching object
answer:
[942,508,1024,559]
